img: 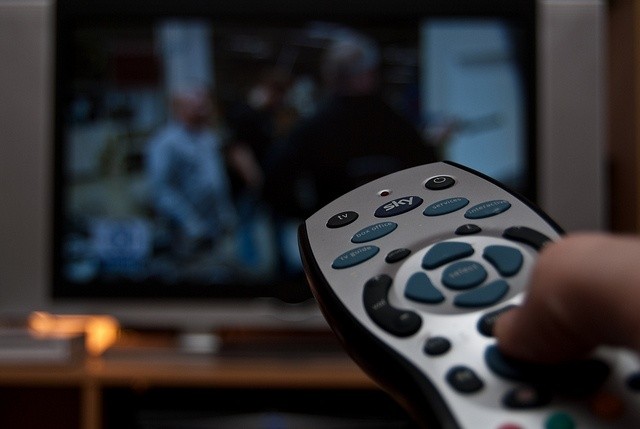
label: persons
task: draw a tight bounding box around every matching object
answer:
[493,232,640,363]
[149,88,239,244]
[235,33,455,295]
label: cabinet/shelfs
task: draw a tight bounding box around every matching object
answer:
[0,360,380,427]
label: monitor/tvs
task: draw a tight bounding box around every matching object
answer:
[37,0,609,361]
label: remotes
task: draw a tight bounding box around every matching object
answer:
[298,159,637,428]
